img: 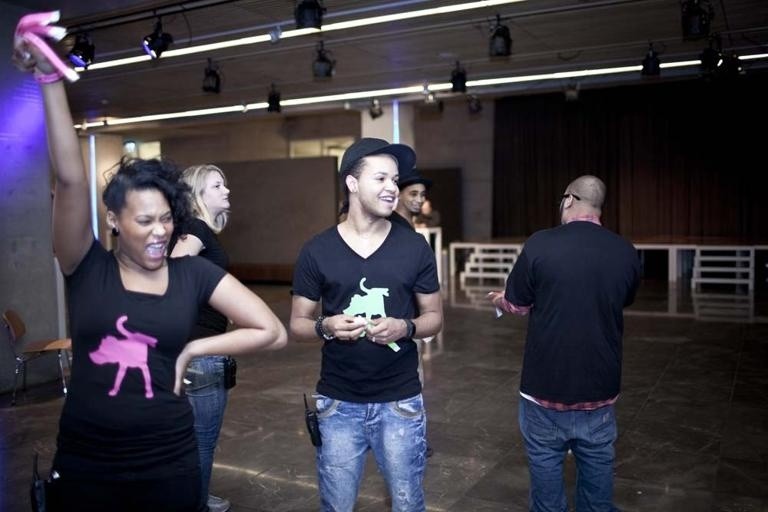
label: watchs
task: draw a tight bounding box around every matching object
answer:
[403,318,416,340]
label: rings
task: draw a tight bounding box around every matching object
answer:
[371,337,376,342]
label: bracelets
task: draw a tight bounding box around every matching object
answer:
[315,317,335,340]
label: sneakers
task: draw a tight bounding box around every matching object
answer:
[207,494,231,512]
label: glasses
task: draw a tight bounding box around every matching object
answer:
[559,191,580,201]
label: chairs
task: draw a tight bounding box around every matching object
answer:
[3,309,73,399]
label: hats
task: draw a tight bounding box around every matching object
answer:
[340,137,416,180]
[397,169,434,192]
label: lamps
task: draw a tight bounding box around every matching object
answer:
[448,59,466,94]
[561,77,581,102]
[69,28,98,71]
[311,39,336,82]
[142,15,173,61]
[679,2,715,45]
[700,32,746,77]
[201,57,226,96]
[370,97,384,118]
[292,1,328,34]
[487,10,513,64]
[640,40,669,80]
[265,84,283,114]
[466,94,484,115]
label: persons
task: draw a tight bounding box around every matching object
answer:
[487,175,642,512]
[291,139,445,512]
[169,162,230,510]
[12,32,286,510]
[393,171,427,231]
